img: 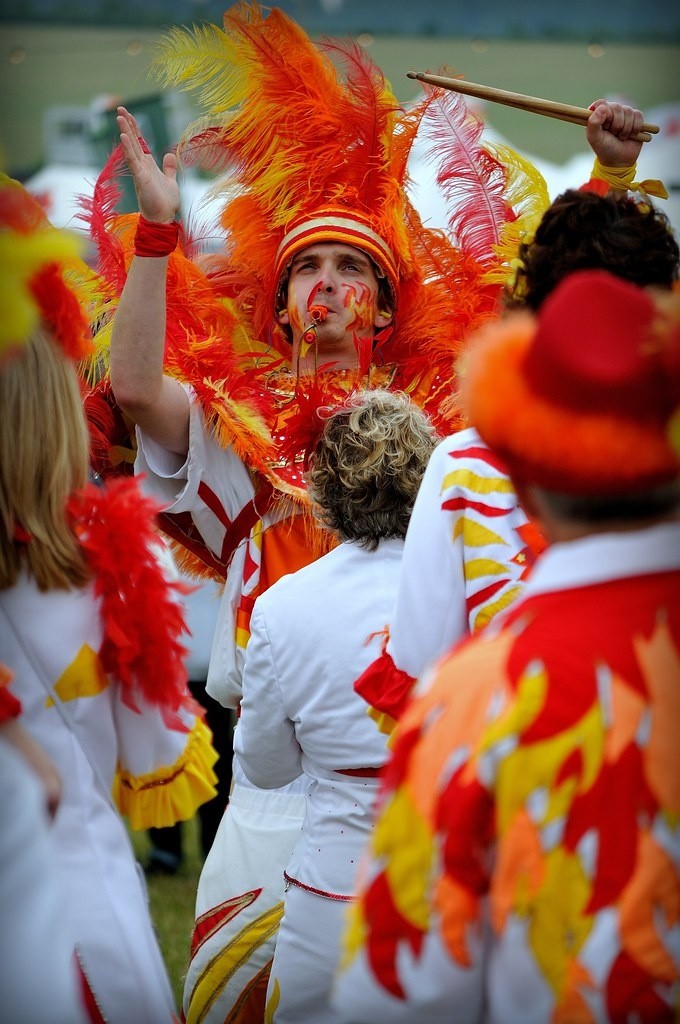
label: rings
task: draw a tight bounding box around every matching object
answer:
[137,136,151,154]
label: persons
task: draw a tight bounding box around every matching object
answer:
[0,168,216,1024]
[134,521,231,880]
[232,388,445,1024]
[109,98,648,725]
[385,189,679,671]
[331,271,679,1024]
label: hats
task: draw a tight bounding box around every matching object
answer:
[453,271,680,487]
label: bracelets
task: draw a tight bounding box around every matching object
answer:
[133,214,179,257]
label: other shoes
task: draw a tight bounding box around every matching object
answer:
[145,849,186,874]
